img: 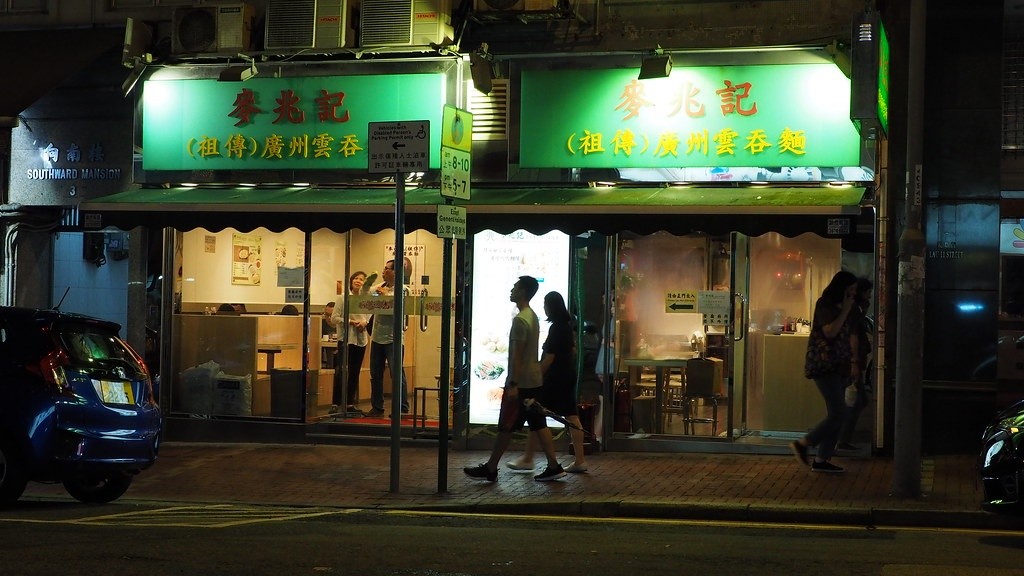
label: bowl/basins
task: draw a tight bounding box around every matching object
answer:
[770,325,784,335]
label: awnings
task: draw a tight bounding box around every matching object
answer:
[78,184,872,239]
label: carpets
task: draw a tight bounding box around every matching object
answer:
[341,417,439,427]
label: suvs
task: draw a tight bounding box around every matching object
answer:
[0,305,162,507]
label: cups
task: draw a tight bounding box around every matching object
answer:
[323,335,329,341]
[332,334,337,340]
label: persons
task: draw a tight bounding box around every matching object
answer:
[790,271,873,473]
[593,287,651,447]
[214,257,410,418]
[463,275,588,484]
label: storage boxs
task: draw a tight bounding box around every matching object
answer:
[686,357,724,396]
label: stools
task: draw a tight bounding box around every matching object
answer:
[619,371,721,436]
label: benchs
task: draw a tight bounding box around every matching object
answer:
[174,312,416,416]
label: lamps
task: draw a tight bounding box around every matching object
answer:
[217,53,258,82]
[468,44,496,96]
[122,56,146,98]
[638,44,674,78]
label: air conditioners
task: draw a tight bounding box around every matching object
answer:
[170,0,255,55]
[360,0,454,49]
[264,0,353,48]
[472,0,560,22]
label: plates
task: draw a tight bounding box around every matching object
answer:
[332,340,337,341]
[255,260,262,269]
[239,249,248,258]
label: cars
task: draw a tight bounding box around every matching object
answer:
[967,335,1024,406]
[976,398,1024,518]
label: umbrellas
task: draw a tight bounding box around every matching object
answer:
[523,397,593,436]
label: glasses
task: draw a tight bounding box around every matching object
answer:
[324,312,332,318]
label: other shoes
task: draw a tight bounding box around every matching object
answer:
[389,410,408,418]
[563,461,587,473]
[507,458,534,474]
[365,406,385,417]
[329,404,360,414]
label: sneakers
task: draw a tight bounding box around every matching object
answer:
[464,463,498,482]
[789,440,810,469]
[812,459,846,474]
[534,465,566,482]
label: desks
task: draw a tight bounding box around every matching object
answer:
[257,343,296,373]
[624,352,697,434]
[321,341,339,369]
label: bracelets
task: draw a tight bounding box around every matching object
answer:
[851,361,859,363]
